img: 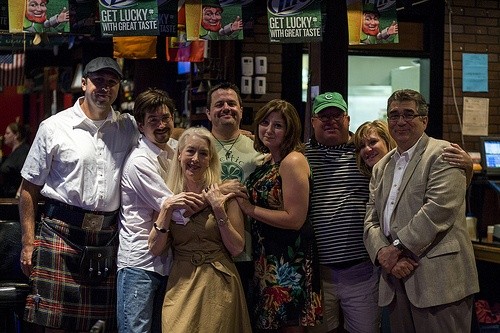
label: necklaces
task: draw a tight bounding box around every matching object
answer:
[211,133,241,158]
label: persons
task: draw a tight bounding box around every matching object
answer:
[236,100,324,333]
[355,120,475,189]
[302,92,382,333]
[0,123,30,197]
[205,85,271,319]
[148,128,252,333]
[363,89,479,333]
[117,87,252,333]
[19,58,138,333]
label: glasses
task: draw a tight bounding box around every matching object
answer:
[143,114,175,125]
[386,112,425,121]
[311,113,345,121]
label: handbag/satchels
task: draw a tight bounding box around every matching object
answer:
[75,244,115,286]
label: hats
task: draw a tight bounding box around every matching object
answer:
[83,56,125,81]
[312,92,348,113]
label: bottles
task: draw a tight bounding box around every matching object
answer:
[466,213,477,241]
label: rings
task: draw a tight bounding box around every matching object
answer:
[205,187,209,193]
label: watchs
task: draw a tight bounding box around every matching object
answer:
[393,239,406,253]
[218,215,228,225]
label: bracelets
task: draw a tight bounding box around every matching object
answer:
[154,222,170,233]
